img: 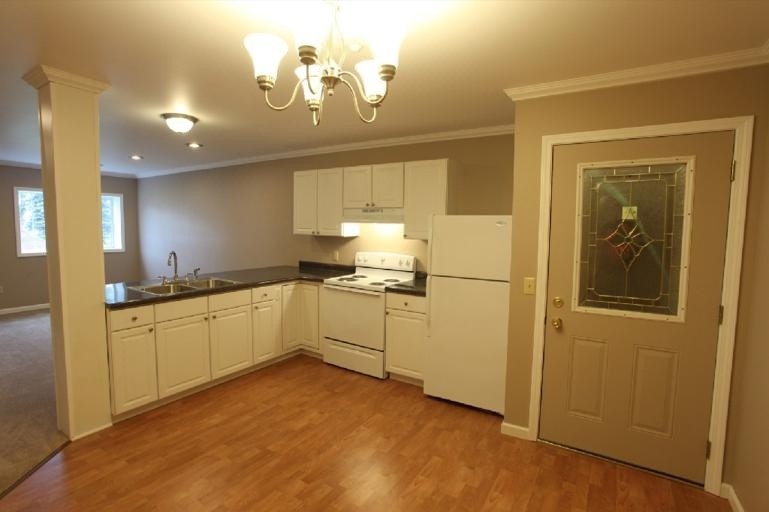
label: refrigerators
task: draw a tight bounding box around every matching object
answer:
[424,214,512,414]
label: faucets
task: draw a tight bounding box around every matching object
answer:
[167,251,178,281]
[193,267,200,279]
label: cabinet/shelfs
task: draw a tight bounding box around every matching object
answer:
[293,160,454,240]
[102,281,322,423]
[386,294,426,388]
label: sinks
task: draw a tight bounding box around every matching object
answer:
[186,278,239,290]
[129,283,200,296]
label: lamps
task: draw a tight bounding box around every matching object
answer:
[243,2,399,128]
[161,113,198,135]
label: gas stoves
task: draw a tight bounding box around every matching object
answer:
[324,267,414,292]
